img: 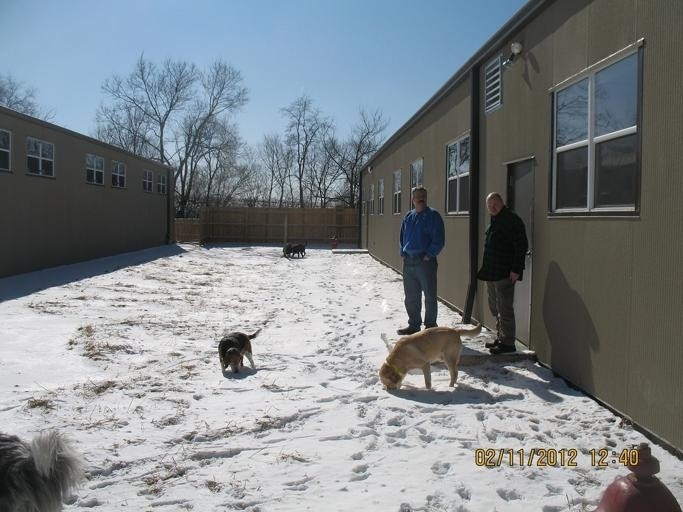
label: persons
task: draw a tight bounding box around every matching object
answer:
[473,190,530,354]
[394,184,444,336]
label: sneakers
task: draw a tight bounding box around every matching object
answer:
[485,338,516,354]
[396,322,438,335]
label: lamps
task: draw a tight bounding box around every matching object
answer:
[502,41,523,69]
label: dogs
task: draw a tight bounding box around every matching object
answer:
[282,240,307,259]
[378,323,482,391]
[0,426,89,512]
[217,328,262,374]
